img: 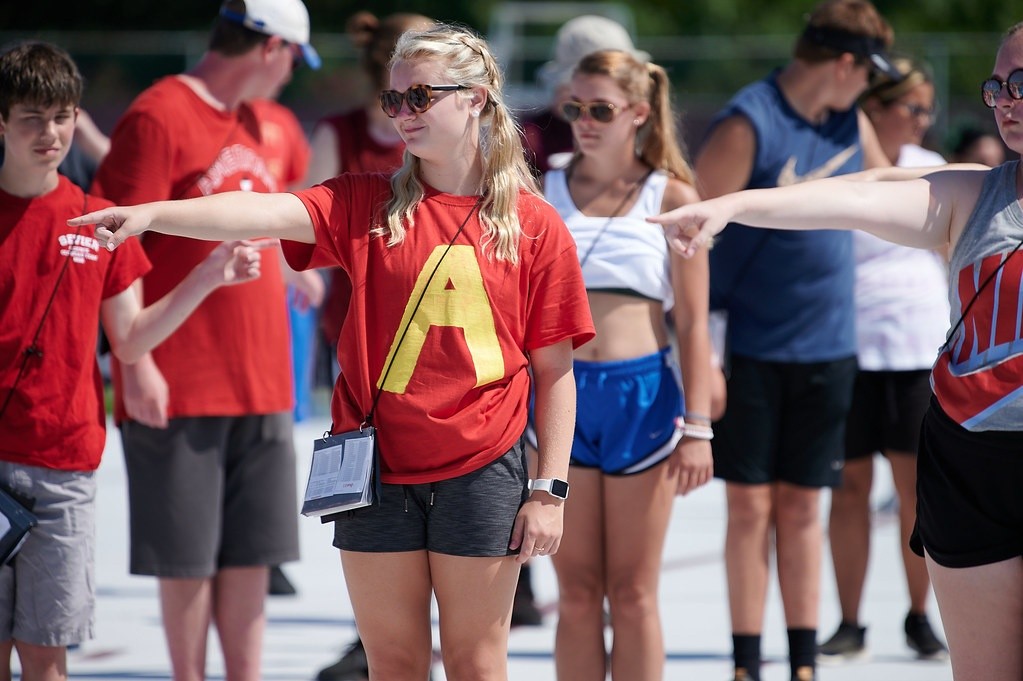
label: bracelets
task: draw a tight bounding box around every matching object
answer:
[682,413,712,422]
[673,416,714,439]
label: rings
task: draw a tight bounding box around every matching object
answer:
[535,546,544,550]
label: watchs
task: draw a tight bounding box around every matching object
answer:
[528,477,569,500]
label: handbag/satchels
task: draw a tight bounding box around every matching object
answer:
[0,482,39,565]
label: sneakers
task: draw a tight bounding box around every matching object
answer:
[319,640,368,681]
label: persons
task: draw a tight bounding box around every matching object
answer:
[534,49,715,681]
[67,16,635,681]
[0,1,321,681]
[644,0,1023,681]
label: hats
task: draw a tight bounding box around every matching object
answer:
[802,28,901,81]
[219,0,322,71]
[555,16,652,72]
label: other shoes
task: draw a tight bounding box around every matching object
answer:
[905,611,949,660]
[820,623,865,655]
[270,567,295,594]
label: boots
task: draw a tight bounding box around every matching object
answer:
[511,564,541,625]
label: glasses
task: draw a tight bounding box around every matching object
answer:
[377,84,471,119]
[557,100,634,123]
[897,99,935,120]
[981,68,1023,108]
